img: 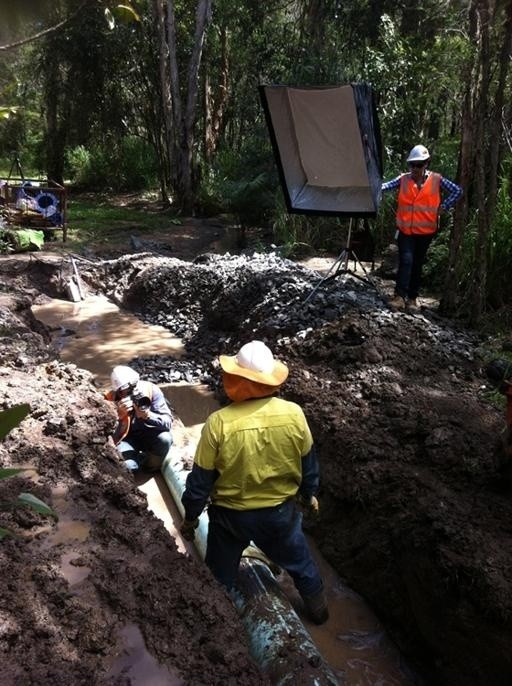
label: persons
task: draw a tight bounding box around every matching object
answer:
[182,340,328,626]
[105,364,174,475]
[382,144,464,312]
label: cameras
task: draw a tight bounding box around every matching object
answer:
[119,379,153,416]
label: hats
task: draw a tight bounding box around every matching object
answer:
[219,341,290,387]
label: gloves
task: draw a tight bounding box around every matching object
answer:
[179,517,199,542]
[298,494,321,527]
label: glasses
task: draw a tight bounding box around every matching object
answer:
[408,162,426,168]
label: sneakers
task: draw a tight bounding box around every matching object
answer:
[387,294,406,312]
[406,296,422,315]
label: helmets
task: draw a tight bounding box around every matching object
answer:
[110,365,140,392]
[406,145,430,162]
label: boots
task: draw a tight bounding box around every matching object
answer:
[299,577,329,625]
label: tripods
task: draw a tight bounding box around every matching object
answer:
[296,217,396,313]
